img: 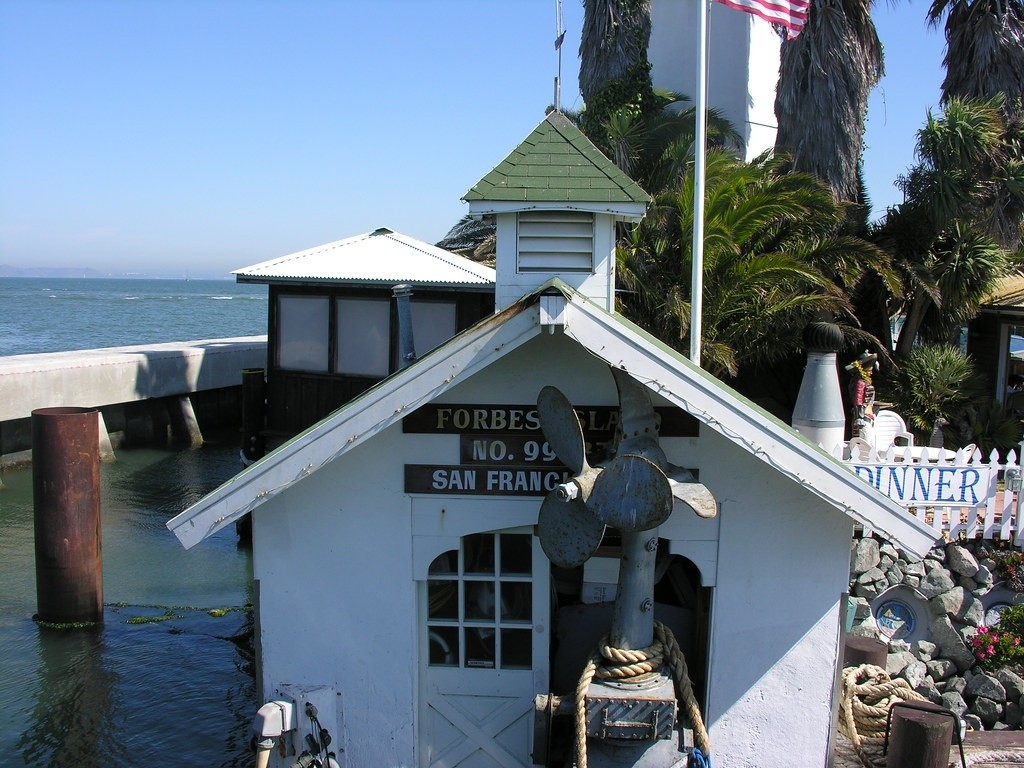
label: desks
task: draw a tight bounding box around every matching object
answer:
[882,444,955,462]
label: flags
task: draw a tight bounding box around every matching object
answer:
[717,0,809,42]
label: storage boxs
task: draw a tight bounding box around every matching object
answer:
[575,558,666,604]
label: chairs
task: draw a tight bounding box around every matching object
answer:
[850,410,976,523]
